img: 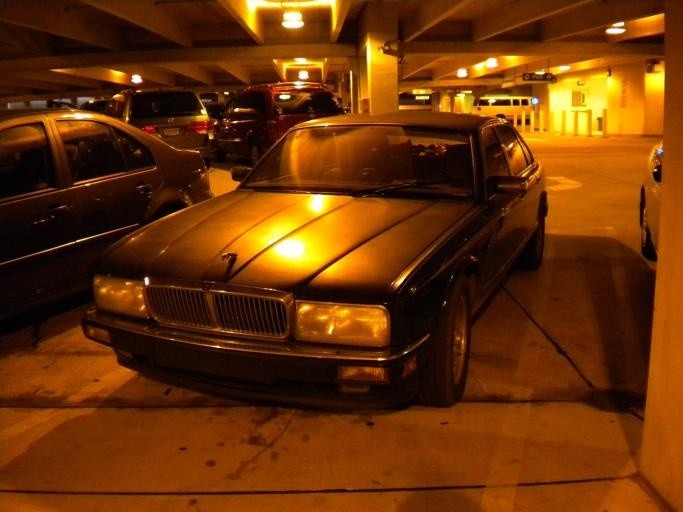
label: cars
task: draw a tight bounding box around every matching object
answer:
[82,114,548,407]
[79,87,229,169]
[0,107,216,330]
[640,138,662,258]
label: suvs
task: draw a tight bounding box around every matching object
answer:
[214,81,344,163]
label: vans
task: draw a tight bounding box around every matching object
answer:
[471,96,536,122]
